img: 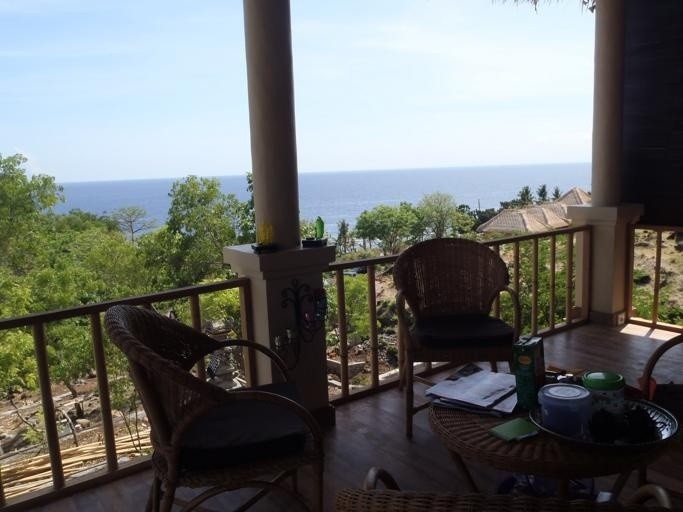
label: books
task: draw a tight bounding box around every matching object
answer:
[425,362,517,417]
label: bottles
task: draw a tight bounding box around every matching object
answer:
[267,224,274,243]
[315,217,325,238]
[259,218,267,244]
[306,217,315,239]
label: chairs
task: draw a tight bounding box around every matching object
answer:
[104,305,324,512]
[333,467,672,512]
[638,335,683,487]
[393,237,521,440]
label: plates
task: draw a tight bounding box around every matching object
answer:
[526,396,679,447]
